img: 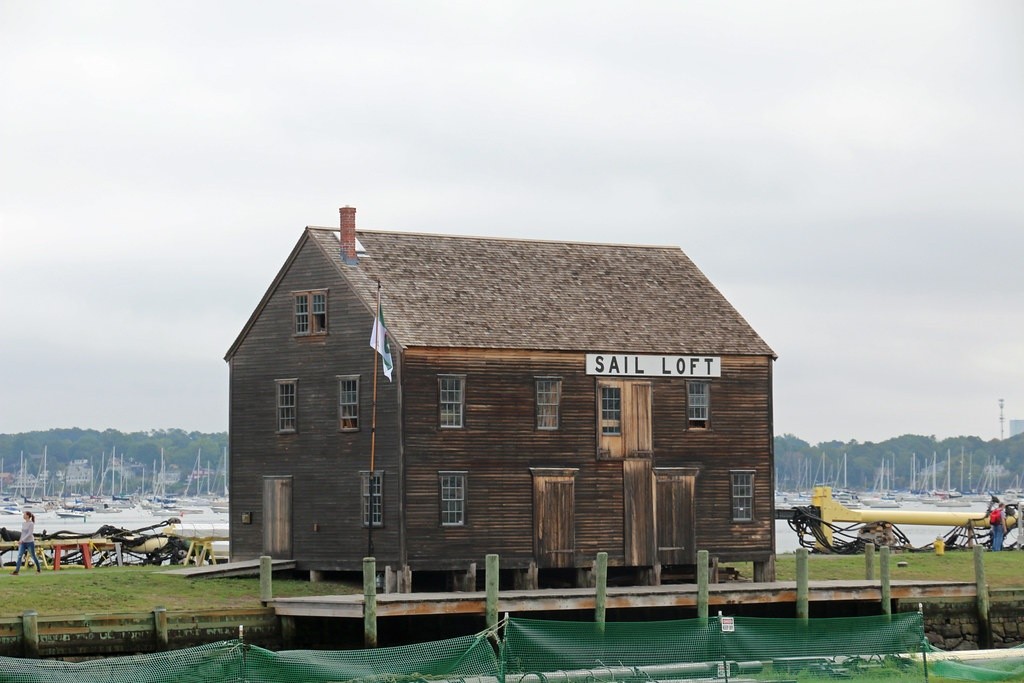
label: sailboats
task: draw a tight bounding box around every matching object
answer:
[775,451,1024,510]
[0,445,230,518]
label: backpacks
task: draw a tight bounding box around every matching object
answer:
[990,509,1001,525]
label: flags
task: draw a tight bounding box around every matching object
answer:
[370,293,394,382]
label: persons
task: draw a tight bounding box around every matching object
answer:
[990,503,1006,551]
[9,511,40,575]
